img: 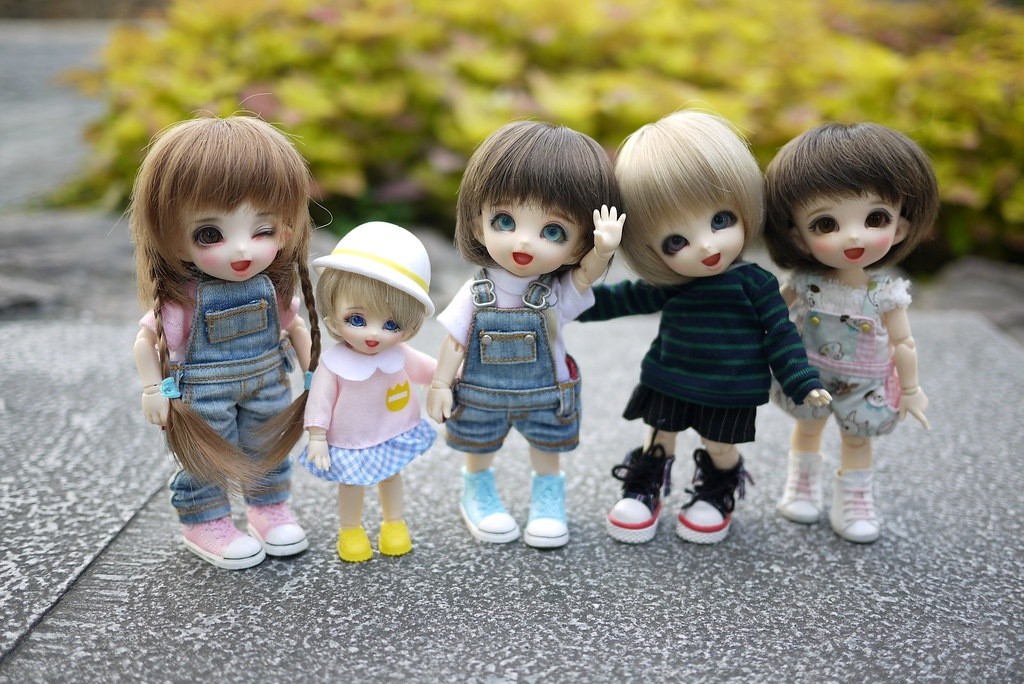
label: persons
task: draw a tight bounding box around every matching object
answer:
[580,109,833,544]
[130,107,325,570]
[424,120,628,548]
[763,123,943,540]
[297,220,443,563]
